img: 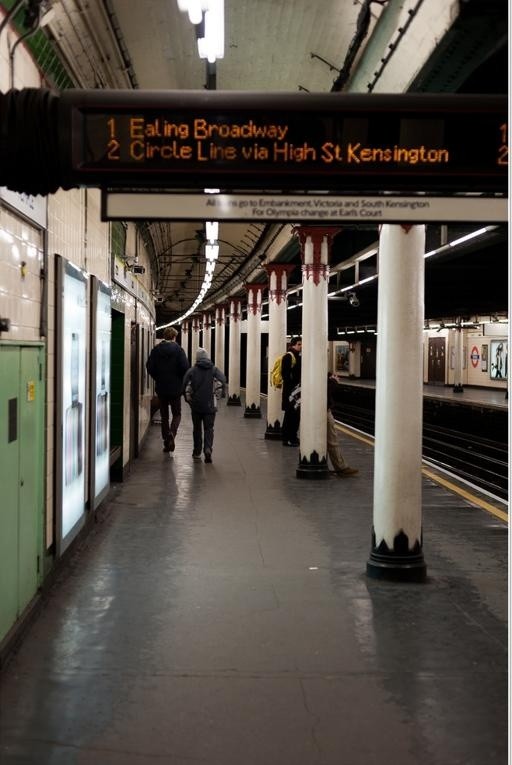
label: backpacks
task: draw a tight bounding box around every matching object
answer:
[270,351,296,389]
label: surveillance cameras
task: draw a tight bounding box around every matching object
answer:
[349,295,360,307]
[154,295,164,302]
[133,265,145,274]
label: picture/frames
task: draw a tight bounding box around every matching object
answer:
[489,339,508,381]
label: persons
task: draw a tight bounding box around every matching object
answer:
[280,336,303,448]
[327,368,357,477]
[146,327,191,454]
[181,346,227,464]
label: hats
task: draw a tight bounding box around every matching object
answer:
[195,348,209,360]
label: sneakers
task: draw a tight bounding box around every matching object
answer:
[337,467,359,476]
[282,437,300,448]
[192,449,212,463]
[162,432,175,452]
